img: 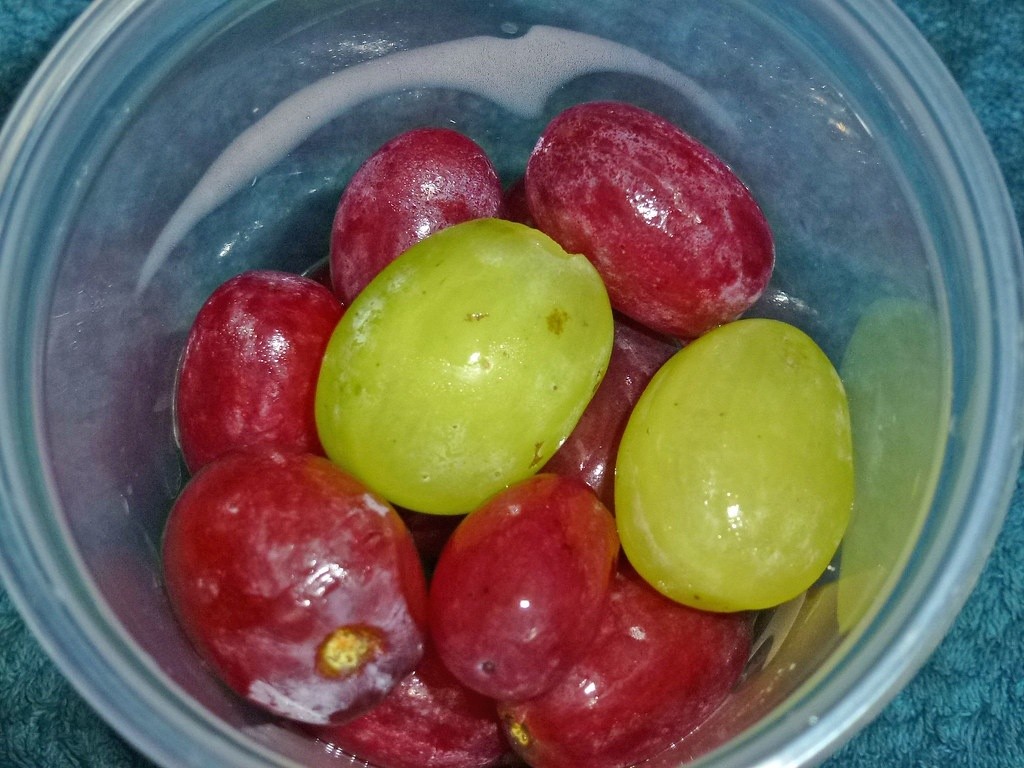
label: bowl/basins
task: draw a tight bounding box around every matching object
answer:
[0,3,1023,768]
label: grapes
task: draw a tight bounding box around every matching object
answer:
[156,101,860,768]
